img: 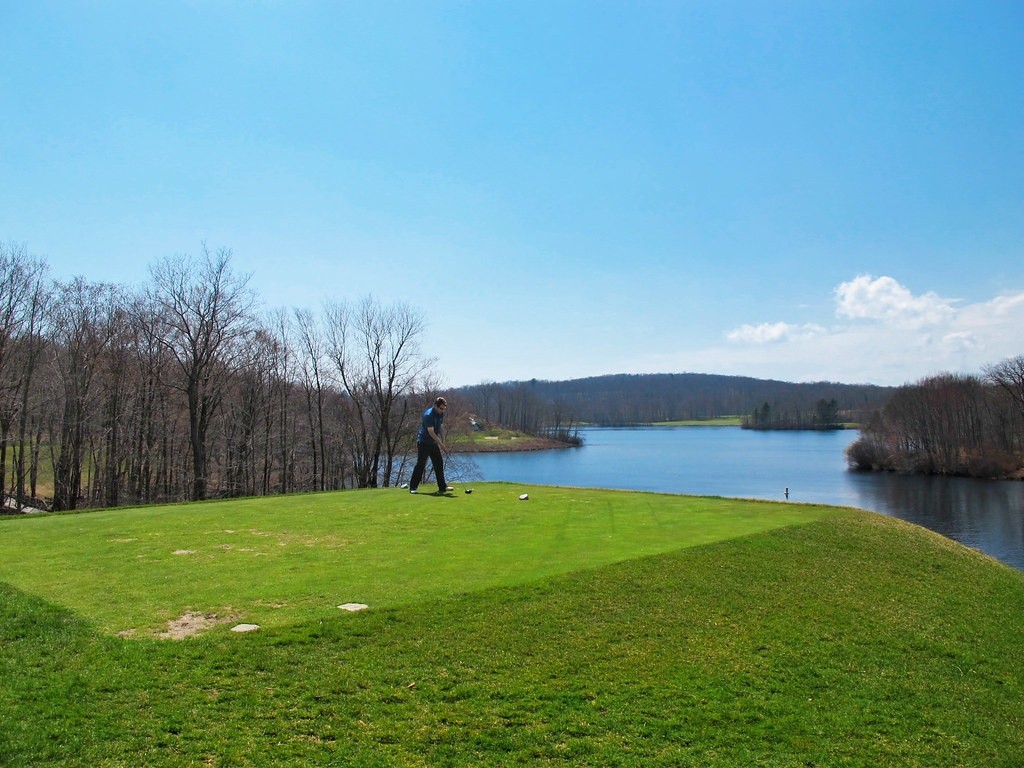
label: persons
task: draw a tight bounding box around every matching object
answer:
[410,397,454,494]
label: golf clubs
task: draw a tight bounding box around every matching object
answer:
[445,451,472,493]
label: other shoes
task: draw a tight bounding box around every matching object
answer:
[439,487,453,491]
[410,490,419,494]
